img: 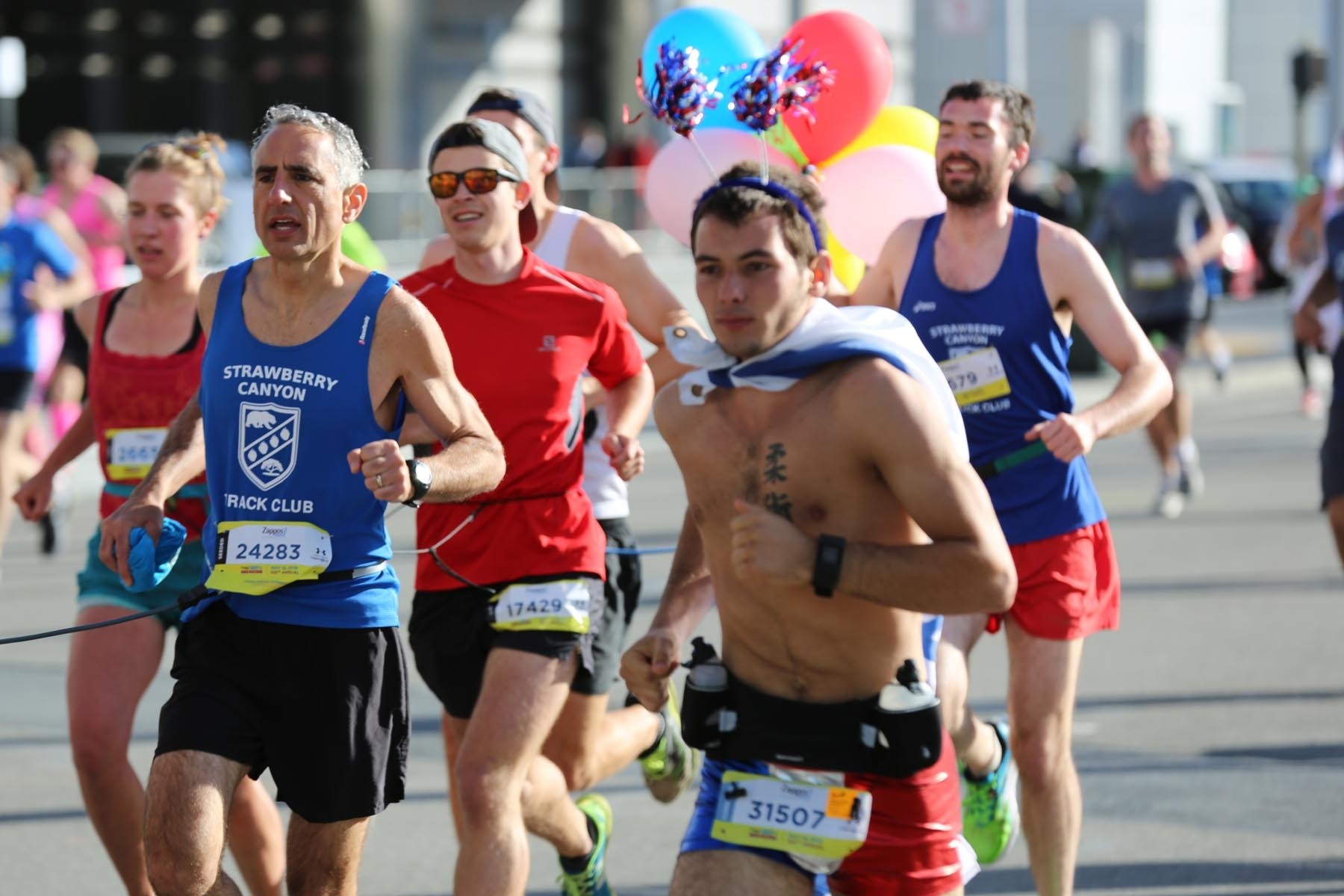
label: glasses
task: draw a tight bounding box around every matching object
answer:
[428,167,520,199]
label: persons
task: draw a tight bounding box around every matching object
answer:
[395,115,656,896]
[422,84,707,803]
[98,100,506,895]
[822,81,1175,896]
[12,131,285,896]
[1007,115,1229,519]
[618,160,1018,895]
[1188,138,1344,575]
[0,126,129,556]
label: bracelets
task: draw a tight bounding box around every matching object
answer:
[809,532,846,598]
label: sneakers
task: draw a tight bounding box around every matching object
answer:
[957,713,1021,865]
[624,676,697,804]
[555,794,617,896]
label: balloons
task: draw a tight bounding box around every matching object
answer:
[640,5,947,297]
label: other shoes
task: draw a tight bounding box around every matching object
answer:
[40,510,54,551]
[1178,453,1203,496]
[1157,488,1181,517]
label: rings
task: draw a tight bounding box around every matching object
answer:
[376,474,382,489]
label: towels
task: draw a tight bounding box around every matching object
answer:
[113,516,188,594]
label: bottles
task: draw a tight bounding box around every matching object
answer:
[880,659,935,711]
[689,637,728,689]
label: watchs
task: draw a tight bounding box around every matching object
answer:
[402,458,432,508]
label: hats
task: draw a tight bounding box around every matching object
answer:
[428,119,538,243]
[467,88,560,202]
